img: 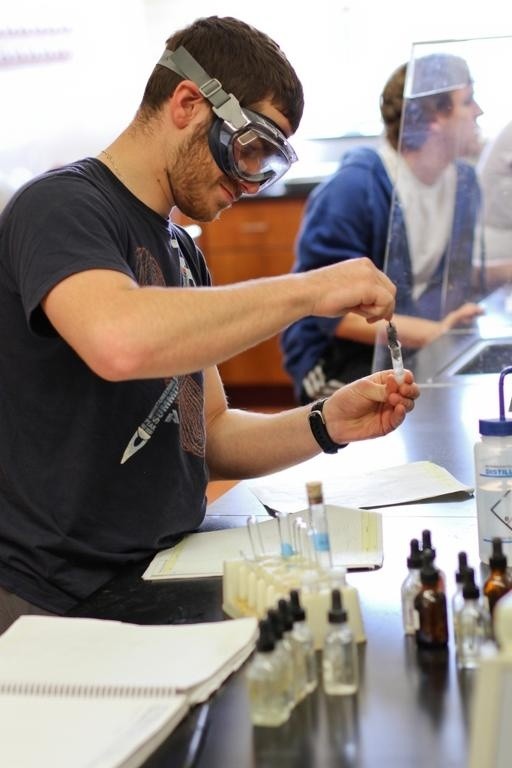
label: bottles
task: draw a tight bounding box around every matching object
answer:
[387,342,406,383]
[245,483,330,579]
[476,418,510,565]
[401,528,512,672]
[246,589,359,727]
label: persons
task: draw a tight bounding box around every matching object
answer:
[282,54,483,406]
[471,122,512,284]
[0,16,420,634]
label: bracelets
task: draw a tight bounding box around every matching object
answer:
[307,399,349,453]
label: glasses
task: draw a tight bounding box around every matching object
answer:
[208,107,299,192]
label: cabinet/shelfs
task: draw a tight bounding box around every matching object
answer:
[168,182,326,400]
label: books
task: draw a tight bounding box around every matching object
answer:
[0,614,261,768]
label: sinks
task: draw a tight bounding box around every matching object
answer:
[435,337,512,375]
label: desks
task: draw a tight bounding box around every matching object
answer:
[59,286,511,768]
